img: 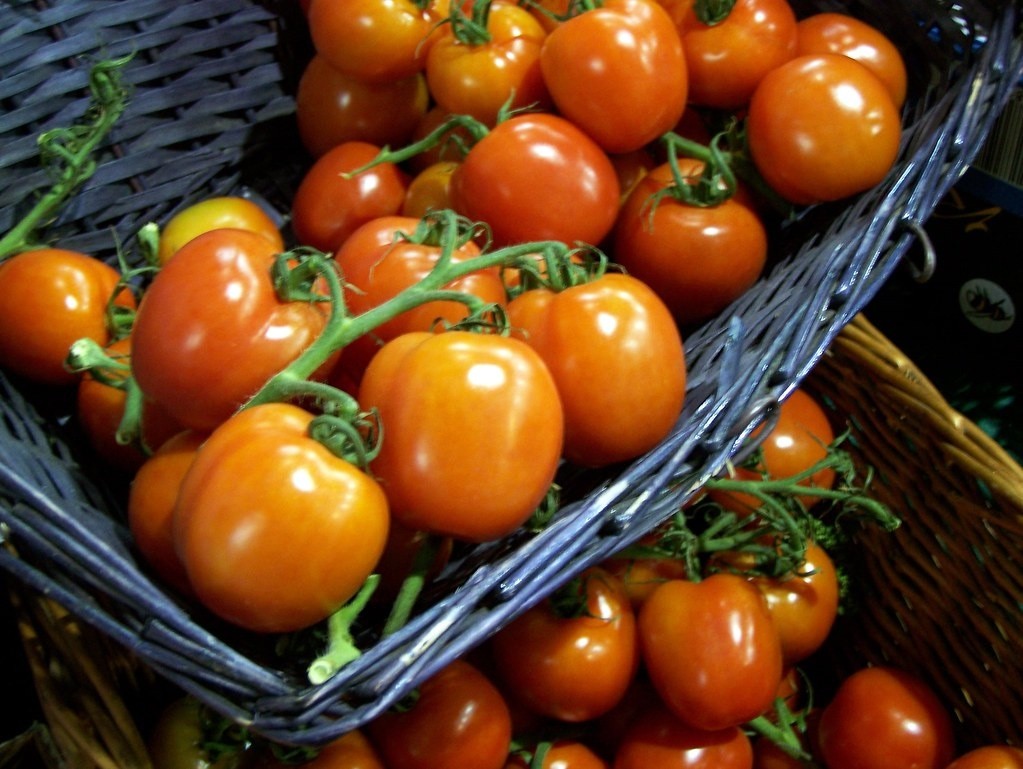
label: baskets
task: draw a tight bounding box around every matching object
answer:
[0,311,1023,769]
[0,0,1023,749]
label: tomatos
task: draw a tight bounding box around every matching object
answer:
[0,0,906,640]
[144,379,1023,769]
